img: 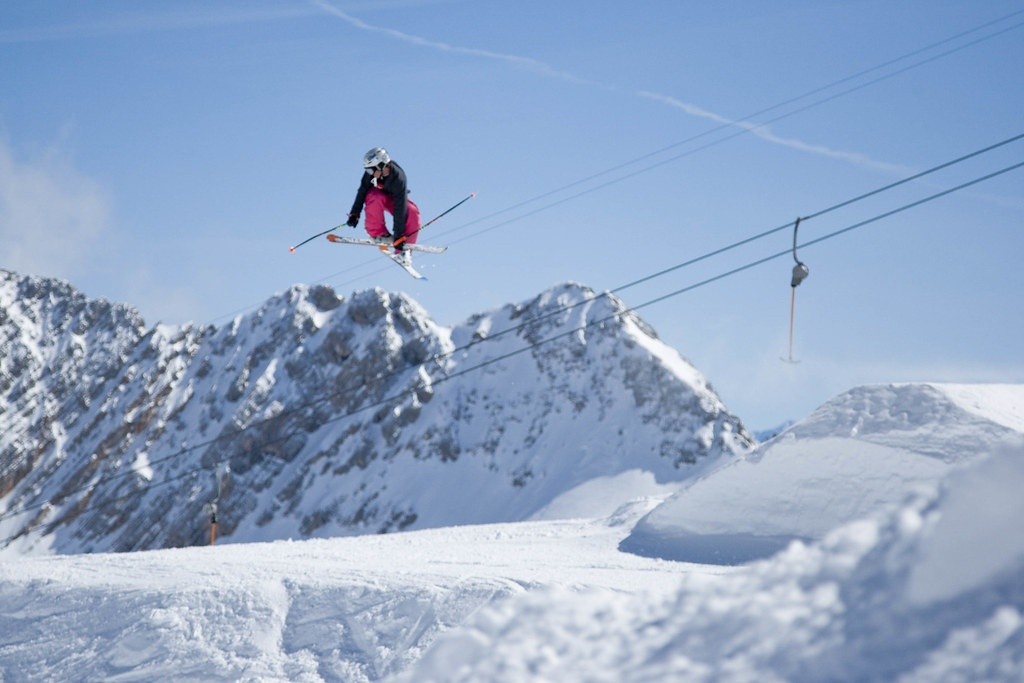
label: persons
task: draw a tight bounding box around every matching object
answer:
[347,148,420,255]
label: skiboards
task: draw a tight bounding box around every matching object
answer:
[326,231,451,280]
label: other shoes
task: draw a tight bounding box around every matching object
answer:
[376,237,393,250]
[395,250,413,264]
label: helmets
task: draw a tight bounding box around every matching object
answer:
[363,147,390,170]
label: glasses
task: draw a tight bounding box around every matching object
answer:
[364,163,384,175]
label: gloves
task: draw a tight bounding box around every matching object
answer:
[393,237,405,250]
[347,215,358,228]
